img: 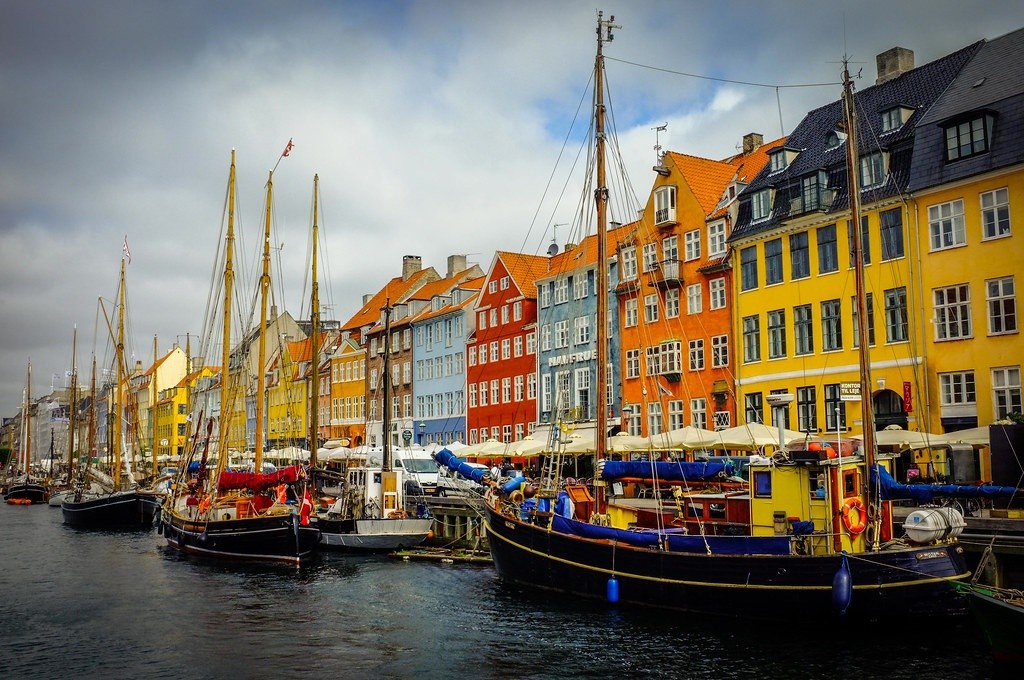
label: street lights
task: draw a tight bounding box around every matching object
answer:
[622,399,630,436]
[431,2,972,614]
[418,420,426,446]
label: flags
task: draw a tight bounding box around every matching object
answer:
[123,238,132,264]
[301,487,312,527]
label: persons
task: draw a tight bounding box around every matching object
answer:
[907,462,920,483]
[814,475,826,499]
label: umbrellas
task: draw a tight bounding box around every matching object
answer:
[229,432,594,478]
[604,421,818,456]
[849,420,990,483]
[129,451,218,462]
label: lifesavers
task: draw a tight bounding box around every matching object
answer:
[386,510,407,519]
[198,494,210,514]
[842,498,868,534]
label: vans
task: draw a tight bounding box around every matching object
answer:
[437,461,496,498]
[365,449,439,495]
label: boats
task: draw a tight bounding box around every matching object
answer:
[161,142,321,571]
[58,256,160,535]
[2,356,48,505]
[318,298,434,551]
[49,427,72,505]
